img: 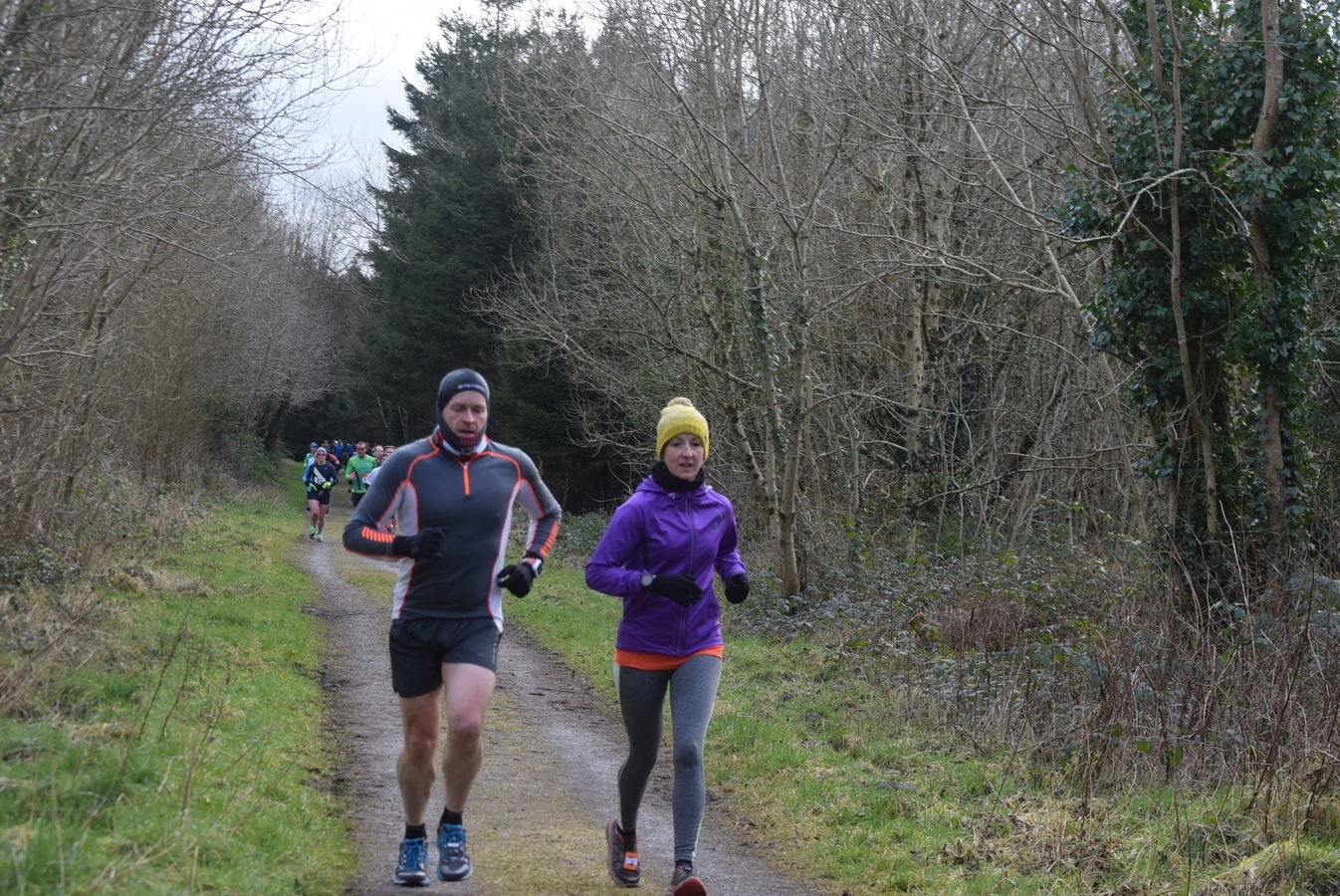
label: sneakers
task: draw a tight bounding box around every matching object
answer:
[669,866,707,896]
[606,820,641,887]
[309,526,316,537]
[436,824,473,882]
[317,533,322,541]
[392,837,430,886]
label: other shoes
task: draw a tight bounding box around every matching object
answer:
[348,489,351,493]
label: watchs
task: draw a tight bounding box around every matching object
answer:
[640,573,657,593]
[520,557,544,578]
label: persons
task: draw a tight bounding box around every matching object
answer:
[303,439,397,509]
[342,368,563,886]
[303,447,339,542]
[584,397,752,896]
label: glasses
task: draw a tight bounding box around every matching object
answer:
[357,448,363,450]
[318,454,325,456]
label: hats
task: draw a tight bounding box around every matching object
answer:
[311,442,318,447]
[437,369,489,413]
[657,397,710,459]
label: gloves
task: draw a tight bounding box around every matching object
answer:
[652,574,703,609]
[350,473,355,480]
[311,484,318,491]
[395,530,446,560]
[724,575,750,604]
[322,482,331,489]
[497,563,535,600]
